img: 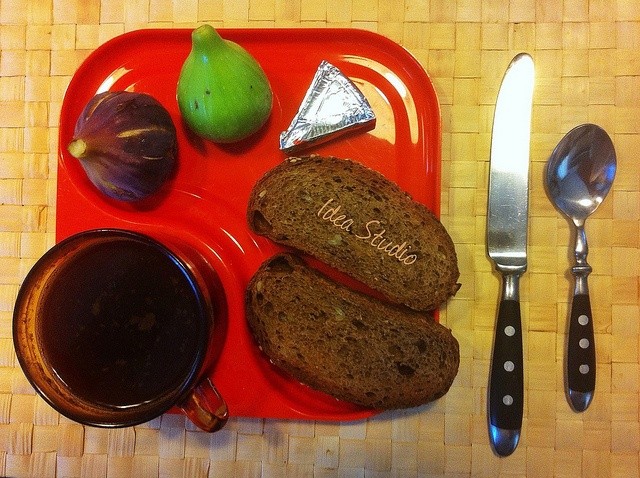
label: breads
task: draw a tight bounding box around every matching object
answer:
[248,252,460,411]
[249,159,461,312]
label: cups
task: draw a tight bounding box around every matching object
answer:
[9,225,227,433]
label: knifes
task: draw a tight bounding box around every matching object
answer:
[486,51,538,458]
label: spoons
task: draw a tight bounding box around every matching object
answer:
[545,123,617,413]
